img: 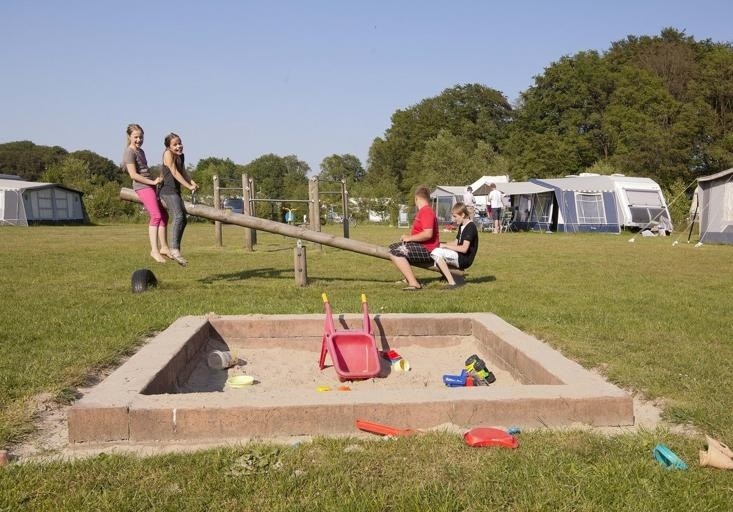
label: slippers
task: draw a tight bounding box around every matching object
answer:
[396,279,420,290]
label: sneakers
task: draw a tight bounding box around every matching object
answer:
[442,283,457,290]
[170,252,189,267]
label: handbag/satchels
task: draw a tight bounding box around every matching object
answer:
[487,205,492,218]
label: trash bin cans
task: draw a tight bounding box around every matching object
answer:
[271,202,285,222]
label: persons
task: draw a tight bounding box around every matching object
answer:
[463,186,476,222]
[430,202,478,288]
[123,123,174,264]
[487,183,505,234]
[387,187,440,290]
[158,132,200,267]
